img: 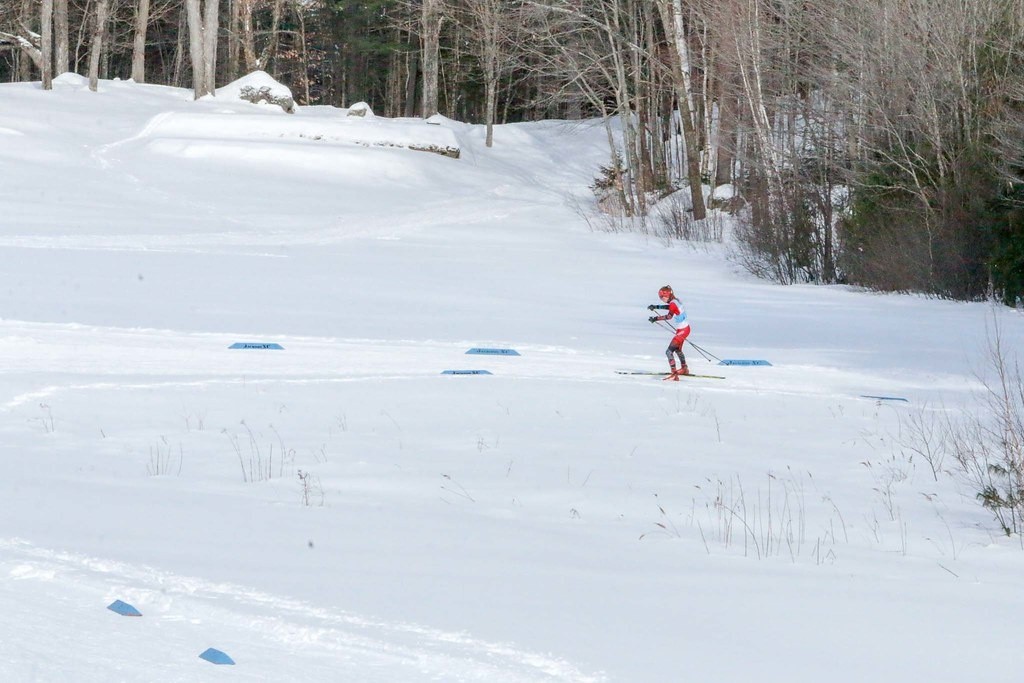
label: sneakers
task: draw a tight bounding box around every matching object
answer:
[677,364,689,375]
[662,373,679,381]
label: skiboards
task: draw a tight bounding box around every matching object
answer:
[613,369,726,380]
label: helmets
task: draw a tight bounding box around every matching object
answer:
[658,285,673,297]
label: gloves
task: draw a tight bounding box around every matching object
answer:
[649,317,657,323]
[648,305,657,310]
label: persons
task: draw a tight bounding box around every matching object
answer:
[647,286,690,381]
